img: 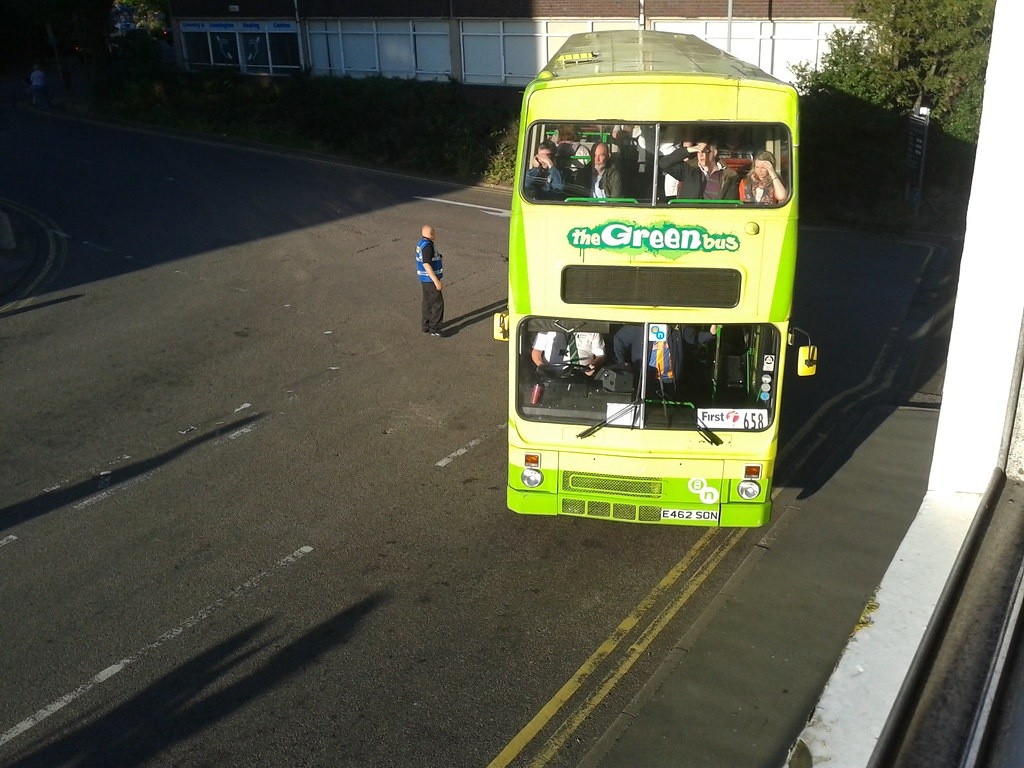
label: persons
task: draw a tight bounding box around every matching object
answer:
[526,122,743,206]
[614,323,722,396]
[415,224,444,338]
[531,329,606,385]
[739,152,789,205]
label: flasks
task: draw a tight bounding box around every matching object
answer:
[530,384,542,405]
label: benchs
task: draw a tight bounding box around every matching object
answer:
[676,152,753,199]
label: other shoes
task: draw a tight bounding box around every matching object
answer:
[422,329,430,333]
[430,330,448,337]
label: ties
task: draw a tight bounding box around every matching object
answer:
[655,340,665,379]
[565,331,580,370]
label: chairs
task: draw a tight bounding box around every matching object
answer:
[709,353,744,405]
[548,125,621,201]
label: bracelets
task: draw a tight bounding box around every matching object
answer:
[772,176,779,181]
